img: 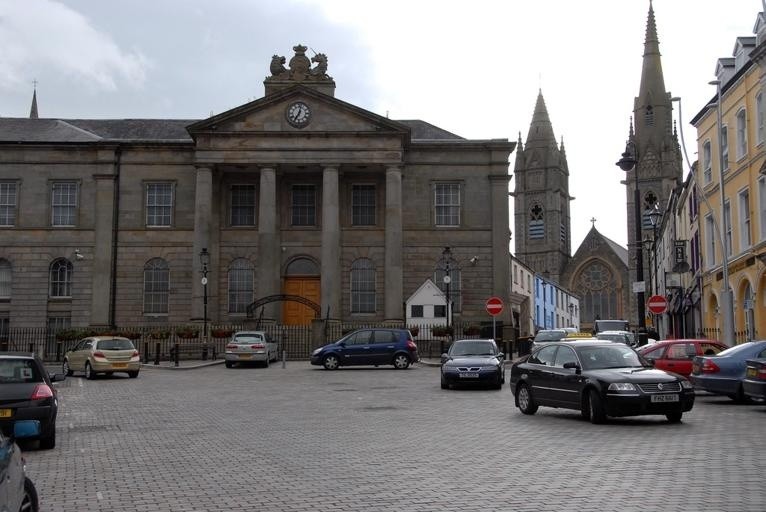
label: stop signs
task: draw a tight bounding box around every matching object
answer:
[486,297,504,316]
[645,294,668,316]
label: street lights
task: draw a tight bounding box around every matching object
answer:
[668,80,735,348]
[441,244,453,328]
[614,138,645,331]
[642,232,654,330]
[648,200,664,337]
[198,246,210,343]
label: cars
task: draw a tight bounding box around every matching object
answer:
[629,337,730,379]
[56,334,143,383]
[529,317,636,353]
[221,329,281,371]
[0,348,58,453]
[741,355,766,407]
[686,337,766,404]
[437,338,505,390]
[508,337,698,428]
[0,429,39,512]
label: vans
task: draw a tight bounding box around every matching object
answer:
[307,326,421,371]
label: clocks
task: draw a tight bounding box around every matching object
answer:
[284,99,312,129]
